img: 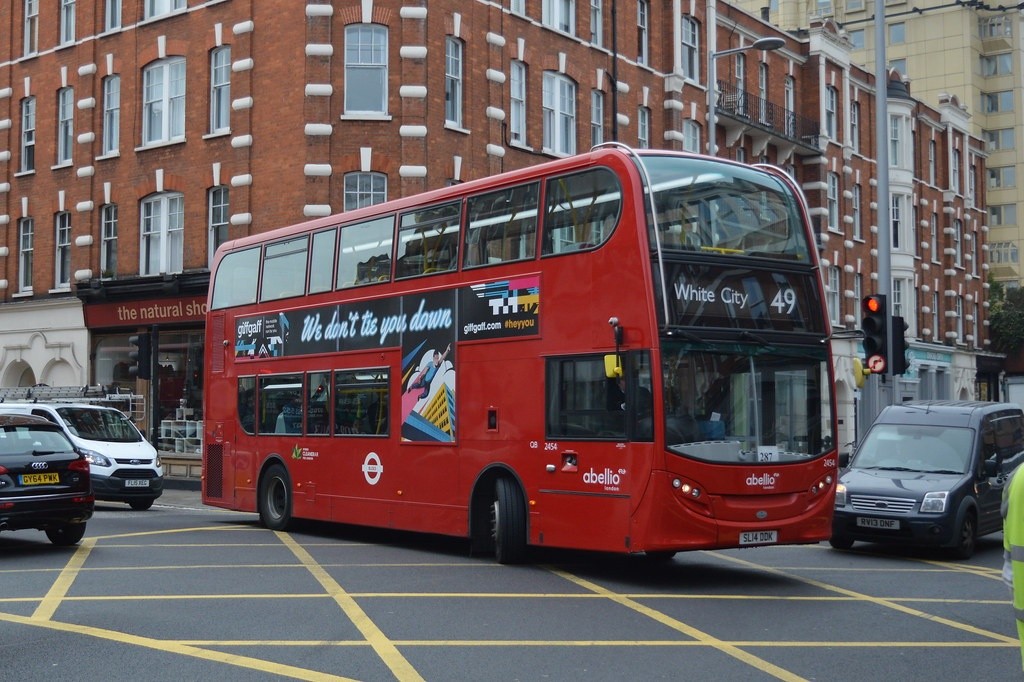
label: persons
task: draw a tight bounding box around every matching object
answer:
[1001,462,1024,671]
[612,378,650,430]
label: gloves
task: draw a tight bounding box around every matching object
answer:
[1002,559,1014,589]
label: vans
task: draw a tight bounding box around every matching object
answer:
[0,401,165,511]
[833,398,1023,562]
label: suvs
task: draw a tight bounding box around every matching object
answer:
[0,414,94,545]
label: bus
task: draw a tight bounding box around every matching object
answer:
[203,143,872,568]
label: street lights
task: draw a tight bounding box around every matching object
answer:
[706,37,786,245]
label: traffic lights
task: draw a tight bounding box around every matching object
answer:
[892,317,911,376]
[861,295,883,354]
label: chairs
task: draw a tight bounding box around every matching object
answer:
[258,389,392,436]
